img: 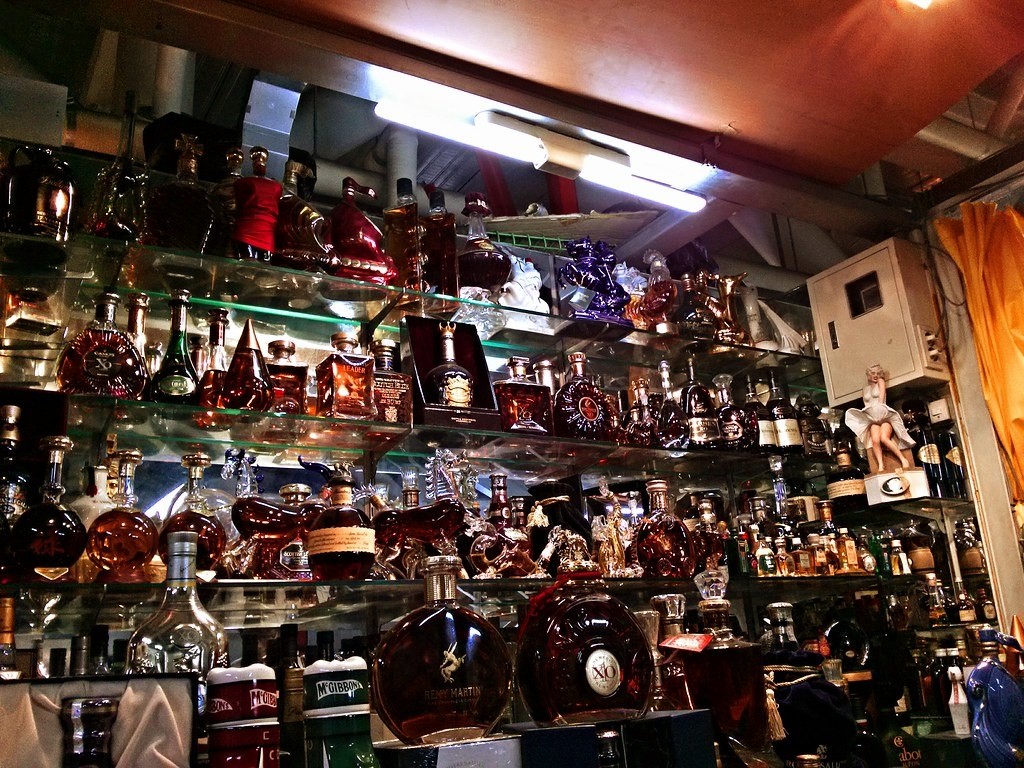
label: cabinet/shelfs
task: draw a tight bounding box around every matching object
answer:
[0,141,1001,768]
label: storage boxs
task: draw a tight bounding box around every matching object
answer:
[500,721,596,768]
[372,733,522,768]
[645,708,717,768]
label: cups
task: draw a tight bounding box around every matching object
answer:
[822,659,844,687]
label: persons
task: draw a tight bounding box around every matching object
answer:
[844,364,916,474]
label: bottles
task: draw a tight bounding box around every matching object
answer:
[0,107,1024,768]
